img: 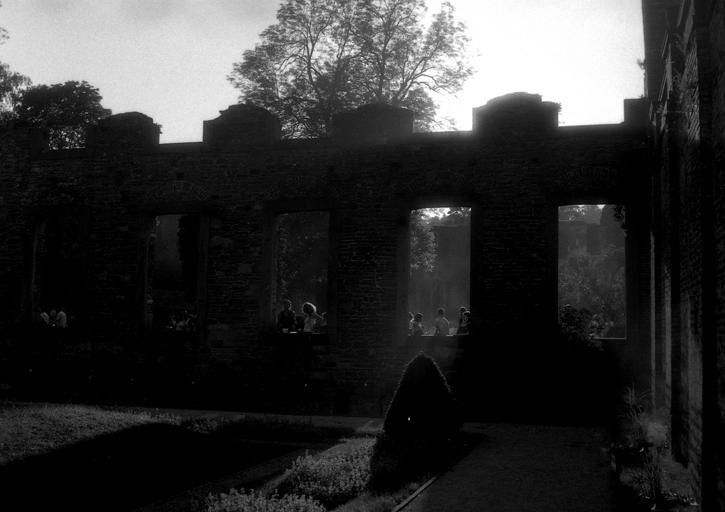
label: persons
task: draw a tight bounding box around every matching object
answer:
[458,307,467,333]
[54,307,68,329]
[435,308,449,335]
[460,311,470,333]
[277,300,296,329]
[50,309,57,326]
[411,312,424,335]
[302,301,320,332]
